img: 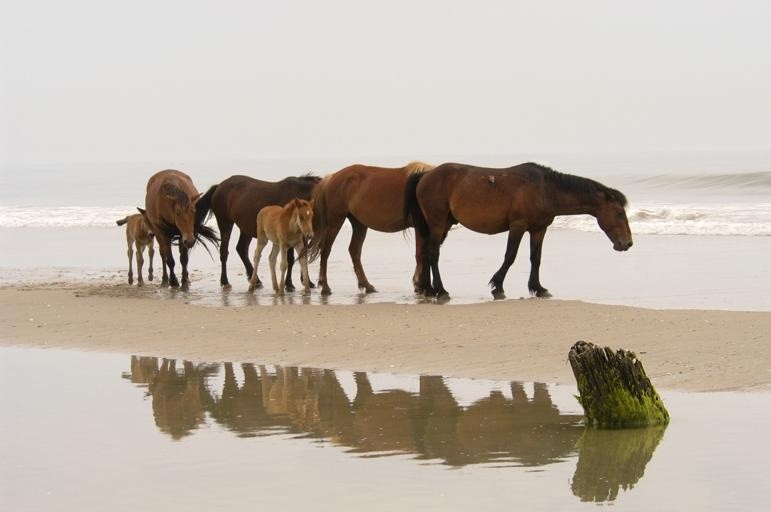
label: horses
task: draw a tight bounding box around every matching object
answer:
[144,167,201,292]
[399,161,634,300]
[311,161,434,298]
[248,198,313,297]
[115,207,156,288]
[193,172,323,291]
[121,356,588,468]
